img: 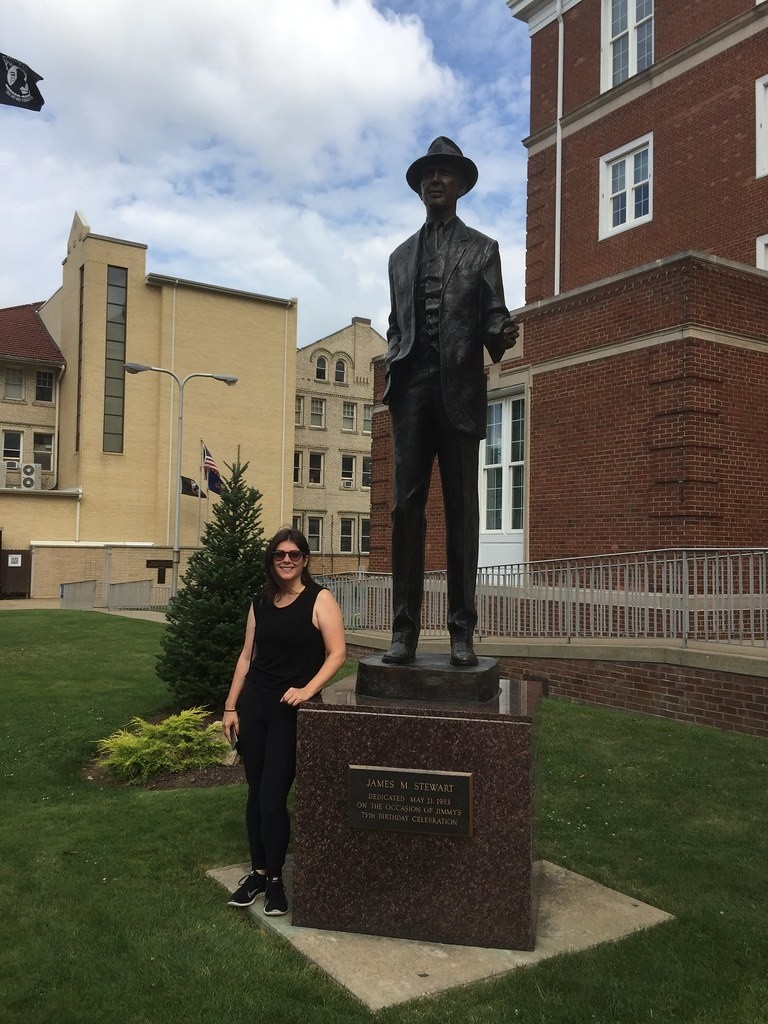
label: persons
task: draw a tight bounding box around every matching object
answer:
[223,529,346,916]
[382,136,520,667]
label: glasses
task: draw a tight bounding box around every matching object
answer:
[271,550,307,562]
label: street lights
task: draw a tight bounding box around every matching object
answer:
[122,360,241,616]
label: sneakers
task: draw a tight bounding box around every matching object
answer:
[227,871,268,906]
[263,874,289,915]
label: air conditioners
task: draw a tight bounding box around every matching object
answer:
[6,461,18,470]
[21,476,41,490]
[21,463,42,477]
[343,481,353,488]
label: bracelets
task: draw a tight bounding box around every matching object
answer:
[223,709,236,712]
